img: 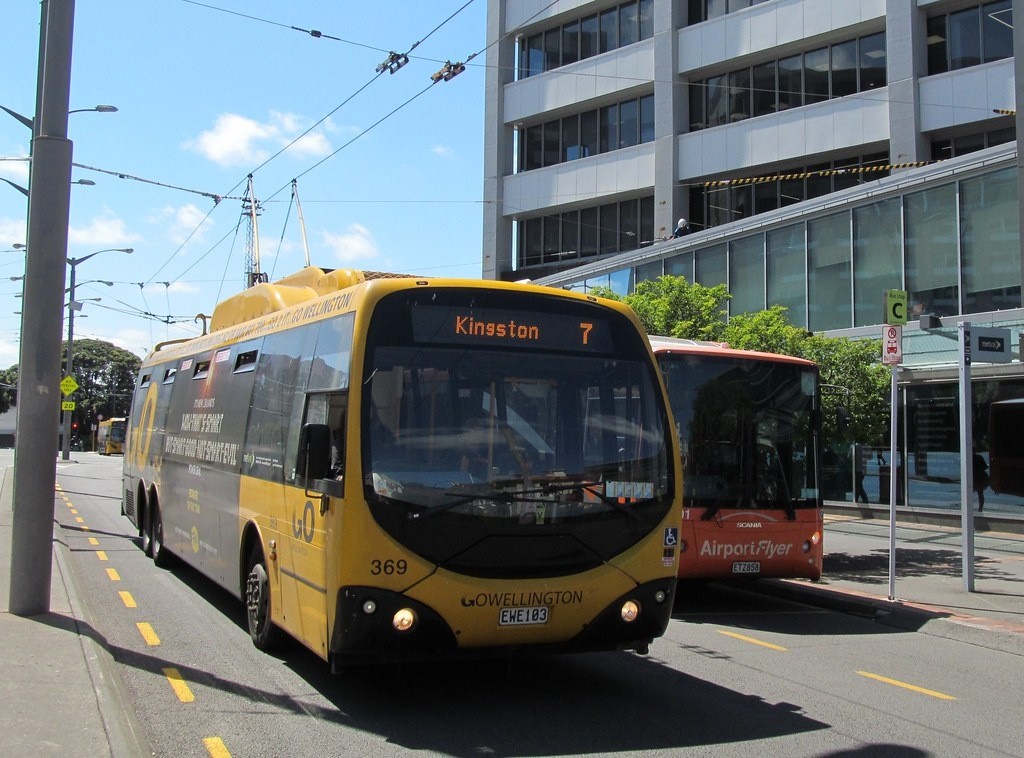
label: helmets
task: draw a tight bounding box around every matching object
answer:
[678,218,687,228]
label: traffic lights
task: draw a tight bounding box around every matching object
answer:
[72,424,78,428]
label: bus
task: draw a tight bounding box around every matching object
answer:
[584,335,852,590]
[98,418,126,456]
[120,267,685,671]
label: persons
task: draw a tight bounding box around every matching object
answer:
[670,218,692,240]
[319,395,402,482]
[969,445,990,512]
[847,429,873,506]
[450,416,512,472]
[876,432,888,466]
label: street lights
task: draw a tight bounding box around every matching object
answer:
[63,315,88,320]
[64,298,102,307]
[29,105,118,157]
[13,244,134,460]
[11,276,113,294]
[71,179,96,185]
